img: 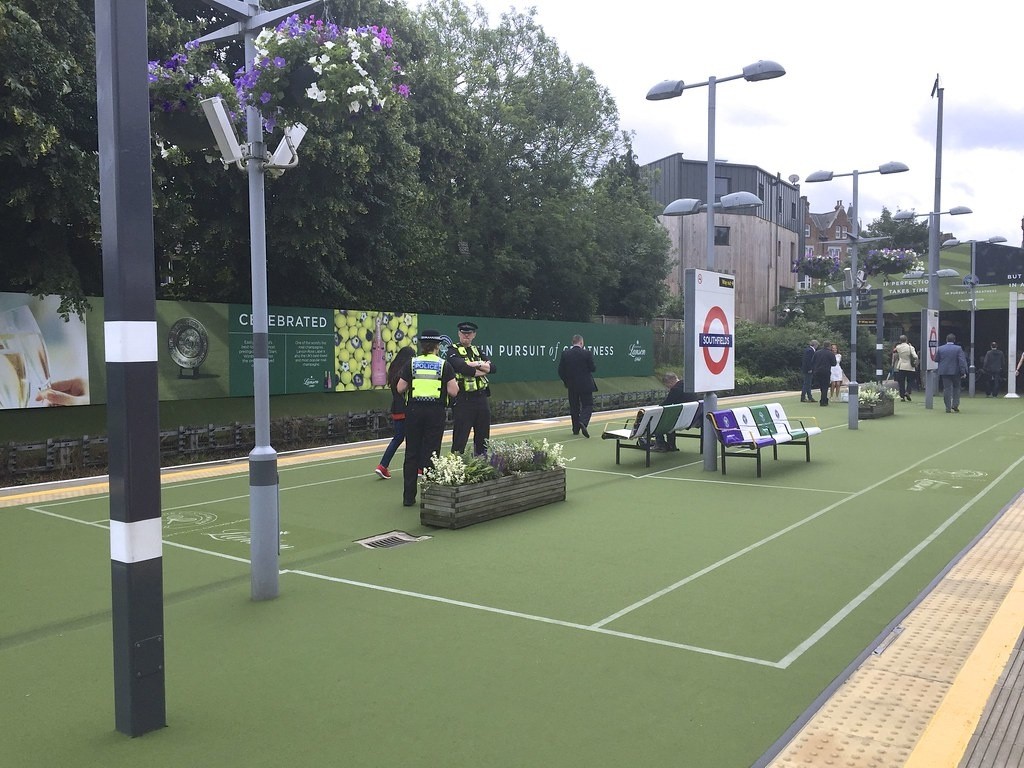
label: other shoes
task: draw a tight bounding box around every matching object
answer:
[946,410,951,413]
[905,392,911,401]
[404,499,416,506]
[649,442,677,452]
[810,398,817,402]
[829,396,840,402]
[951,405,960,411]
[901,397,904,402]
[801,399,807,402]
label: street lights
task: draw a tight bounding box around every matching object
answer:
[804,161,911,431]
[892,205,973,410]
[941,236,1008,399]
[646,59,787,471]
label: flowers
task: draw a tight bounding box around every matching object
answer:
[419,437,576,493]
[865,248,925,274]
[859,380,900,407]
[147,36,240,123]
[792,254,841,274]
[233,12,408,133]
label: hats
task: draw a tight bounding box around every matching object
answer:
[419,330,443,342]
[456,321,479,334]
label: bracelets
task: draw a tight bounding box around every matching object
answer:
[478,361,481,367]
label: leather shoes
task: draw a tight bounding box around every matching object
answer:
[578,421,590,438]
[573,431,579,434]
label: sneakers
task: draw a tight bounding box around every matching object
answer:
[417,468,424,477]
[375,465,392,479]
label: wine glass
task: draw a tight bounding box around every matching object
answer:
[0,305,55,408]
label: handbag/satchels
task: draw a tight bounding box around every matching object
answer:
[910,353,918,368]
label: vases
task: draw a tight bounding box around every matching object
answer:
[882,261,907,274]
[858,397,895,420]
[290,65,329,120]
[804,267,830,279]
[421,468,565,530]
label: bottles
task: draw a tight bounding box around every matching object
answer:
[371,321,387,386]
[324,370,331,389]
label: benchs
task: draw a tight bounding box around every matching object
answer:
[601,400,705,467]
[705,403,821,478]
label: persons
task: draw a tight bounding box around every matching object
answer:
[1015,352,1024,376]
[935,334,966,413]
[375,346,422,479]
[983,342,1004,398]
[447,322,496,456]
[558,335,598,437]
[650,372,698,452]
[397,330,459,507]
[892,335,918,402]
[801,340,818,402]
[811,341,836,406]
[830,344,843,403]
[35,377,90,405]
